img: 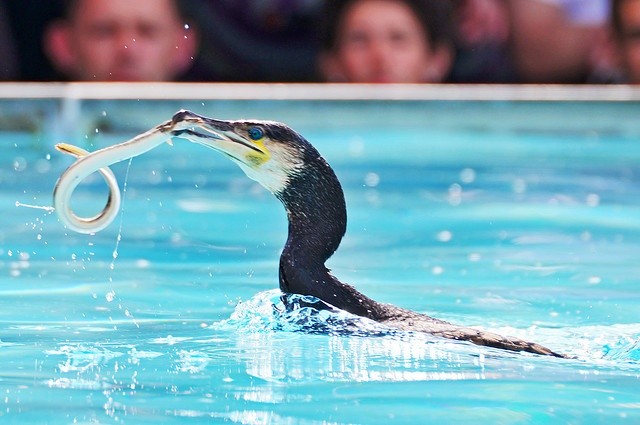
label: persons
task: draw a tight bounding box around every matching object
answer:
[46,1,196,80]
[318,1,454,83]
[611,0,640,83]
[506,2,611,83]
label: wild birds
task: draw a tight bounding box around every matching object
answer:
[170,110,571,357]
